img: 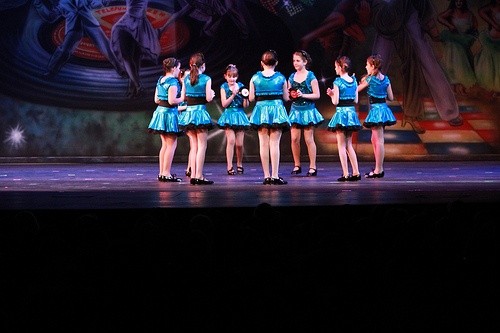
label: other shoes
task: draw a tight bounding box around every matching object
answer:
[307,168,317,177]
[291,166,301,176]
[157,174,176,181]
[263,178,271,186]
[185,172,191,177]
[271,177,288,185]
[364,169,385,178]
[227,167,237,175]
[237,167,243,175]
[162,175,182,182]
[337,173,361,181]
[190,178,195,184]
[195,178,214,184]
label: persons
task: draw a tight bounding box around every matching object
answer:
[289,51,324,177]
[218,64,249,175]
[327,56,361,181]
[146,57,184,182]
[178,69,193,177]
[357,53,397,178]
[0,202,500,332]
[178,53,215,184]
[250,49,290,185]
